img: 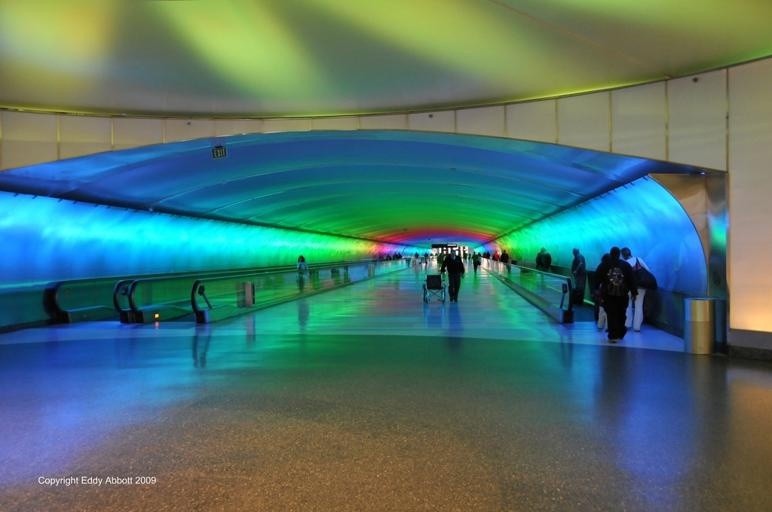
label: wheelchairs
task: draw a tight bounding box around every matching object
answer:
[422,273,446,303]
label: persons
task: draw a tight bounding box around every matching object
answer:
[368,247,552,275]
[570,247,587,307]
[619,245,650,333]
[596,247,639,343]
[598,252,608,332]
[297,255,308,279]
[441,248,465,306]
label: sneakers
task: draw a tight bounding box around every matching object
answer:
[596,321,645,345]
[448,296,459,305]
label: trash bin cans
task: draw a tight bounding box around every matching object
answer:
[684,298,715,355]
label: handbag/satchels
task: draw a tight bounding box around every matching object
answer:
[630,257,658,293]
